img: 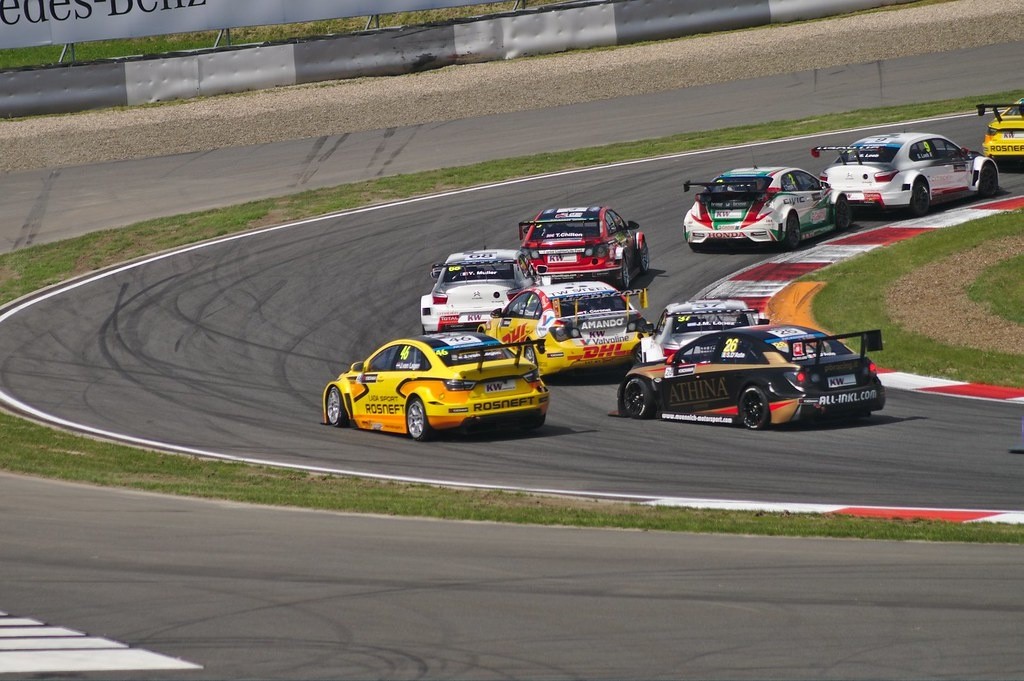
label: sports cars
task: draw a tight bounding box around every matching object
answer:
[475,282,655,378]
[419,247,549,338]
[812,134,1003,215]
[635,301,771,368]
[682,166,854,251]
[322,332,551,443]
[976,98,1024,162]
[616,323,886,431]
[516,204,648,289]
